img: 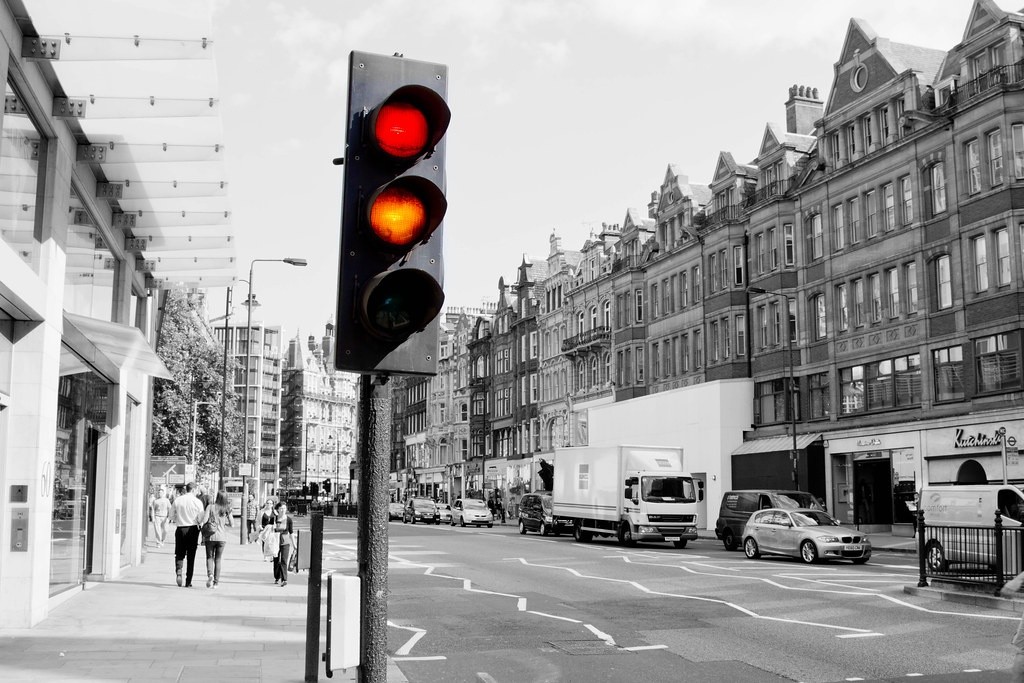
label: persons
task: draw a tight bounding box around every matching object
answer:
[488,495,502,521]
[912,492,919,538]
[257,499,297,587]
[810,497,827,512]
[149,481,234,589]
[247,494,259,545]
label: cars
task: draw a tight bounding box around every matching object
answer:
[387,502,408,522]
[401,497,441,525]
[436,503,452,525]
[742,508,873,564]
[451,498,493,528]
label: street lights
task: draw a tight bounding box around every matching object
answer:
[238,258,309,546]
[746,287,800,488]
[327,428,339,505]
[218,281,264,492]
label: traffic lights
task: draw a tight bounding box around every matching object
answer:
[323,479,330,492]
[312,484,319,495]
[335,51,454,380]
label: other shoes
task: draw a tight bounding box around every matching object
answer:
[176,569,182,587]
[214,582,218,588]
[281,581,287,587]
[206,575,213,588]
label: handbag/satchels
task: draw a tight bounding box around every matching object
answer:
[202,522,215,538]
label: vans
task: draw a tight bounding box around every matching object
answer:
[714,490,830,549]
[919,483,1024,573]
[518,493,573,536]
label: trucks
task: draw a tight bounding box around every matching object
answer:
[550,444,706,552]
